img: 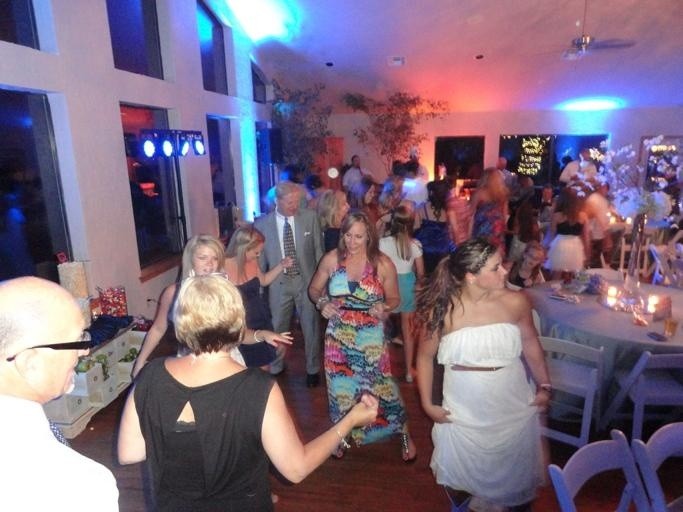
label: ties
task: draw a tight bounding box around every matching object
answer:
[281,217,300,275]
[46,411,72,449]
[358,166,364,178]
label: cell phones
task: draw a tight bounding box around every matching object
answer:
[647,332,667,341]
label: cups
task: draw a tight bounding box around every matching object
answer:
[664,316,679,337]
[562,270,573,285]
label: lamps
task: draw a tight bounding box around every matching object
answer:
[139,128,206,158]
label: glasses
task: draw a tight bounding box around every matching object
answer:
[6,327,95,364]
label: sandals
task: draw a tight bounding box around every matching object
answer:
[399,430,417,465]
[404,366,417,384]
[331,445,346,460]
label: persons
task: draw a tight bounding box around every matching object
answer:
[502,148,683,292]
[1,149,552,511]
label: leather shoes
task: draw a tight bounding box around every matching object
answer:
[306,371,320,389]
[270,360,286,377]
